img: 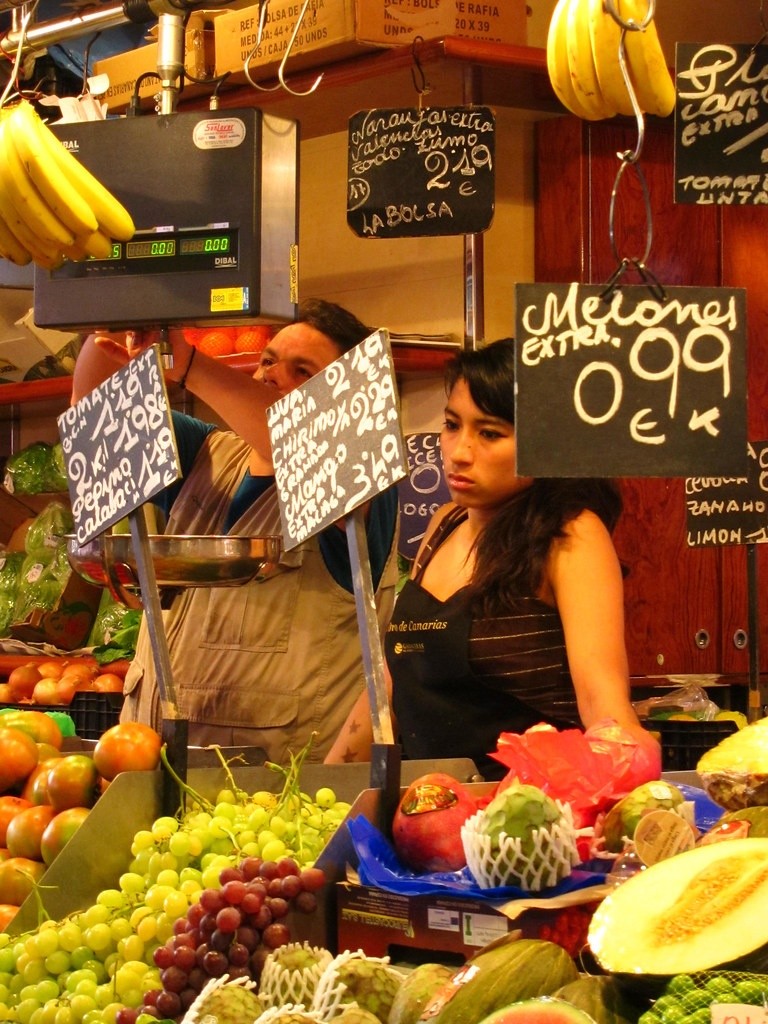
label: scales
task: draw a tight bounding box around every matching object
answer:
[33,108,285,606]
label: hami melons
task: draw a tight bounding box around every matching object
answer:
[580,835,768,975]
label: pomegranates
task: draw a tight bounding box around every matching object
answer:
[1,658,123,707]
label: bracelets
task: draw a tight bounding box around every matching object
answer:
[178,344,197,391]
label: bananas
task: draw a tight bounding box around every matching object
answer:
[546,1,677,121]
[0,98,135,267]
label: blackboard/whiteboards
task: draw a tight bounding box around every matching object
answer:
[348,106,495,239]
[56,343,182,550]
[683,440,768,546]
[266,326,407,553]
[515,282,748,480]
[674,42,768,204]
[397,432,453,558]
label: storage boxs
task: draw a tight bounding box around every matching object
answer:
[213,0,527,87]
[93,26,212,115]
[1,647,768,1024]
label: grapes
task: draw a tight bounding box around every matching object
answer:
[0,730,351,1024]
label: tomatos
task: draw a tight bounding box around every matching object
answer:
[0,706,161,933]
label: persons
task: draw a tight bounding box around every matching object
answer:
[71,298,405,767]
[323,337,650,780]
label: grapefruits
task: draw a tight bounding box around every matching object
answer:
[0,437,144,643]
